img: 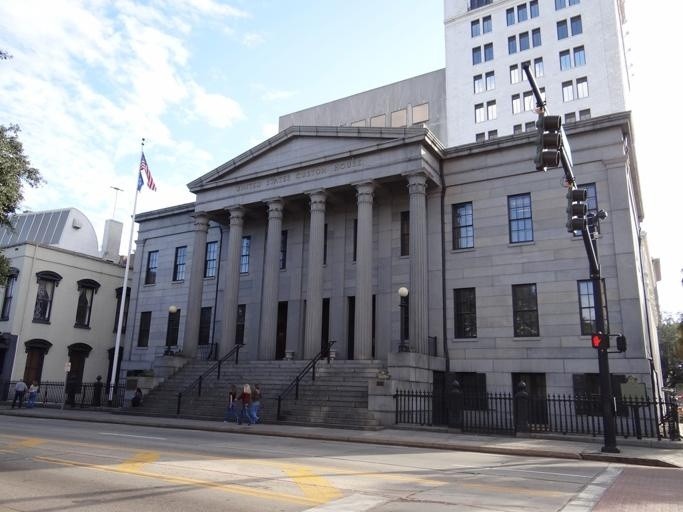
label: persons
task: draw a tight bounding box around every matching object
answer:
[234,383,253,425]
[224,384,238,424]
[132,387,143,407]
[251,383,263,423]
[11,378,28,409]
[25,379,40,408]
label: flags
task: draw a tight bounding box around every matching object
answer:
[137,152,156,192]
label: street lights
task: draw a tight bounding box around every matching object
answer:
[168,305,177,351]
[399,287,408,345]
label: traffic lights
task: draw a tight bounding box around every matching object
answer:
[566,186,586,233]
[534,112,561,172]
[592,334,626,352]
